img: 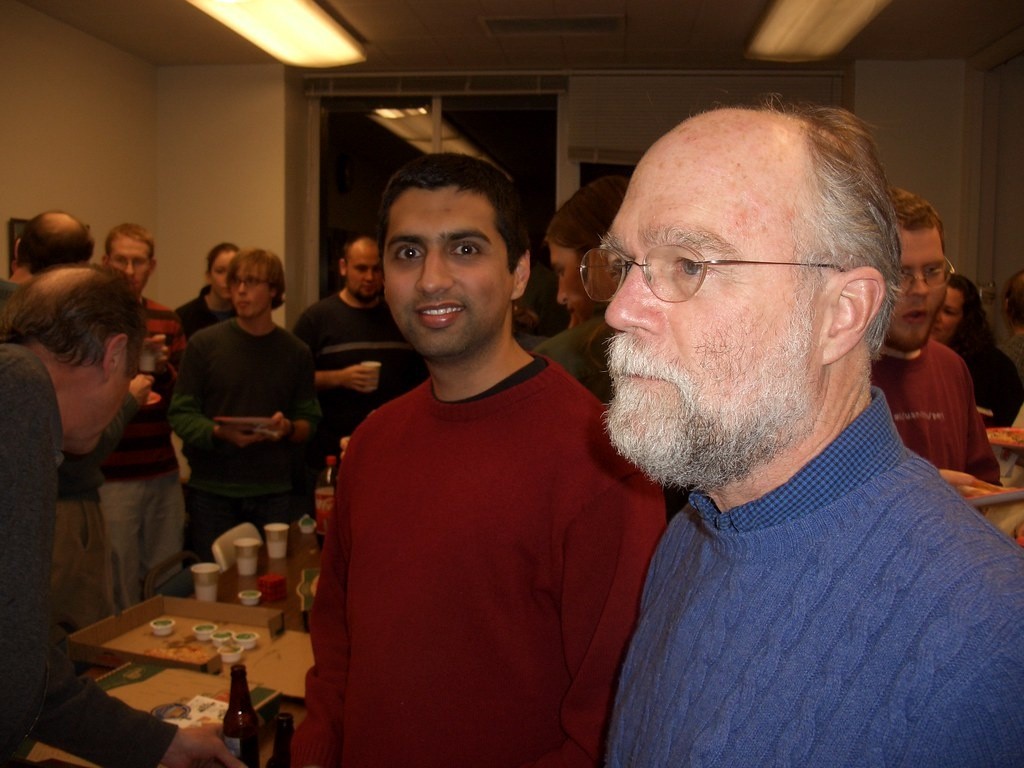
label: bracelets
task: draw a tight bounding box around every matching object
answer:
[281,426,295,441]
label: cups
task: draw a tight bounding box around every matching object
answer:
[265,524,289,559]
[191,563,219,602]
[139,351,156,371]
[361,361,381,390]
[234,538,260,575]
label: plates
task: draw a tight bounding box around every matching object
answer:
[140,390,161,405]
[987,428,1024,447]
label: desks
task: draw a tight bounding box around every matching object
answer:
[20,523,321,768]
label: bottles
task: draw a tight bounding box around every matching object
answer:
[315,455,338,554]
[266,712,294,768]
[221,663,261,768]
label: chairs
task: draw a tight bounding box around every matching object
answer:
[49,459,117,631]
[143,551,203,599]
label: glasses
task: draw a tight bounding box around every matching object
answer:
[897,255,956,293]
[578,247,846,304]
[226,276,270,286]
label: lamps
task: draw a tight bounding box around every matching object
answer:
[187,1,369,69]
[743,0,888,61]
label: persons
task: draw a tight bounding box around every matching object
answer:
[0,177,1024,630]
[289,153,668,768]
[0,262,251,768]
[577,105,1024,768]
